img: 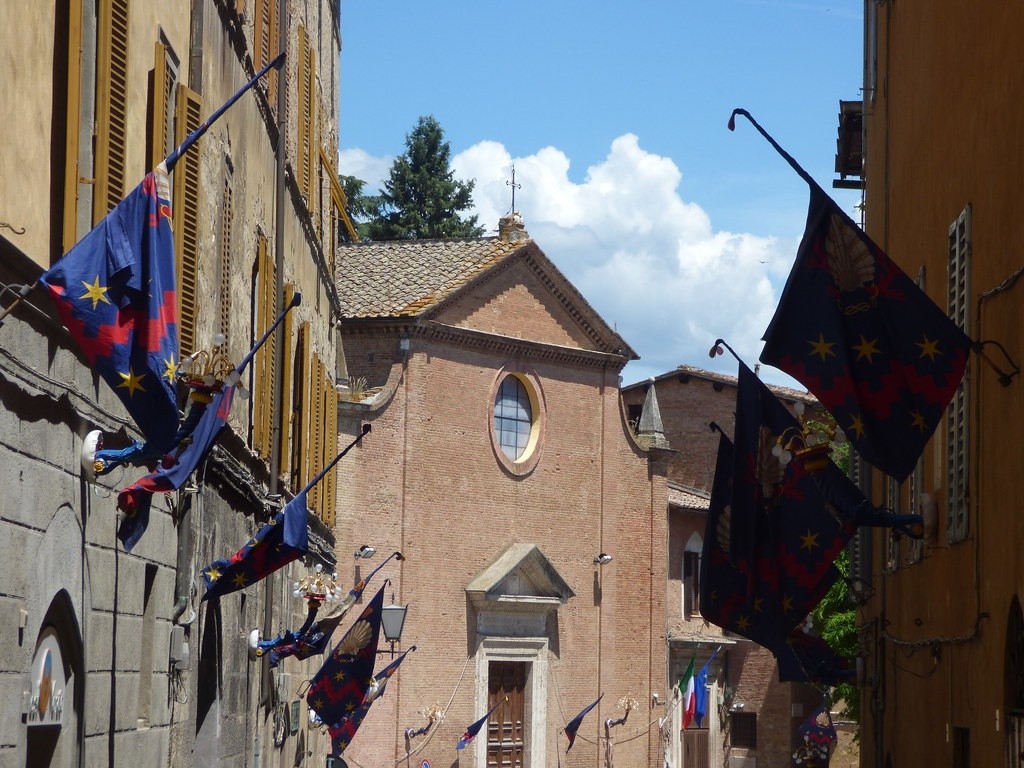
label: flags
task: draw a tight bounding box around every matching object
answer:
[563,694,602,755]
[456,707,494,749]
[38,159,415,759]
[686,180,969,768]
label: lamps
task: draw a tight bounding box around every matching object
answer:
[593,552,612,564]
[307,675,379,730]
[791,736,827,768]
[772,400,937,543]
[81,331,251,477]
[604,691,639,728]
[354,544,376,559]
[405,699,444,738]
[785,612,867,690]
[376,590,408,658]
[248,563,342,660]
